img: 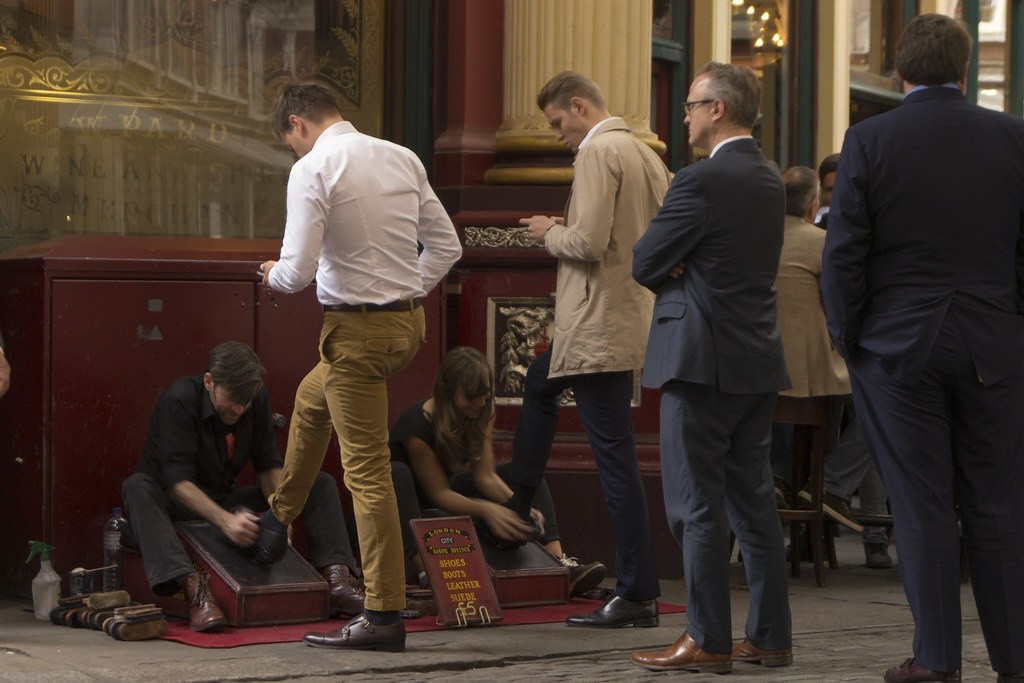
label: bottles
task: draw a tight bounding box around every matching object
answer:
[103,508,130,593]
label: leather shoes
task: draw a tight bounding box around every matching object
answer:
[185,572,228,631]
[628,632,732,674]
[883,657,961,683]
[476,502,526,549]
[228,502,288,564]
[321,563,366,609]
[302,613,407,652]
[567,596,659,627]
[996,675,1024,683]
[732,639,793,667]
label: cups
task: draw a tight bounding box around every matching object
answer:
[69,574,94,597]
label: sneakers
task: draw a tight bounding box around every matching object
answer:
[863,538,895,569]
[797,483,865,533]
[556,551,607,598]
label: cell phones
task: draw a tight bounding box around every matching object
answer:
[256,270,265,277]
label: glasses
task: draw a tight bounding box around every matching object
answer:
[682,99,727,114]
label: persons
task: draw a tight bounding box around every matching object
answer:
[260,81,462,649]
[475,71,672,627]
[779,154,892,564]
[823,13,1024,683]
[389,346,607,596]
[120,342,415,631]
[631,63,796,671]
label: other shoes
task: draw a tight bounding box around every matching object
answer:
[419,574,433,591]
[774,479,791,508]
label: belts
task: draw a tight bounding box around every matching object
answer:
[324,297,421,312]
